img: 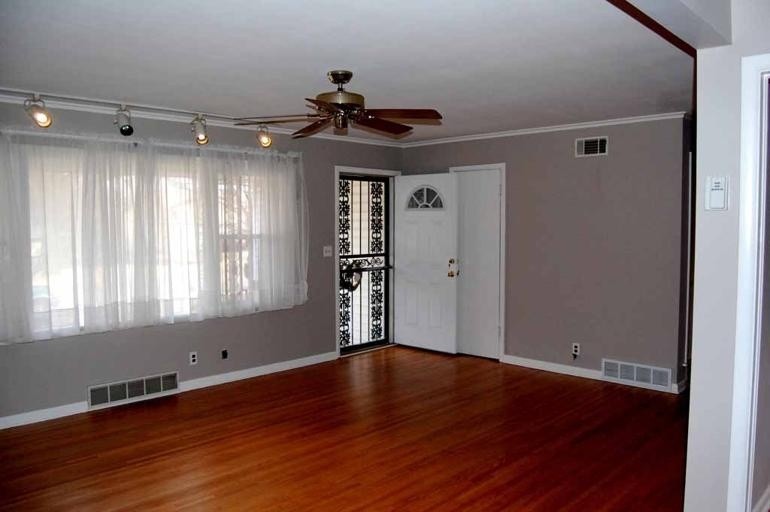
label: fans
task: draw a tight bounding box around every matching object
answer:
[231,70,444,137]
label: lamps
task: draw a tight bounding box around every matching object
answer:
[22,90,273,148]
